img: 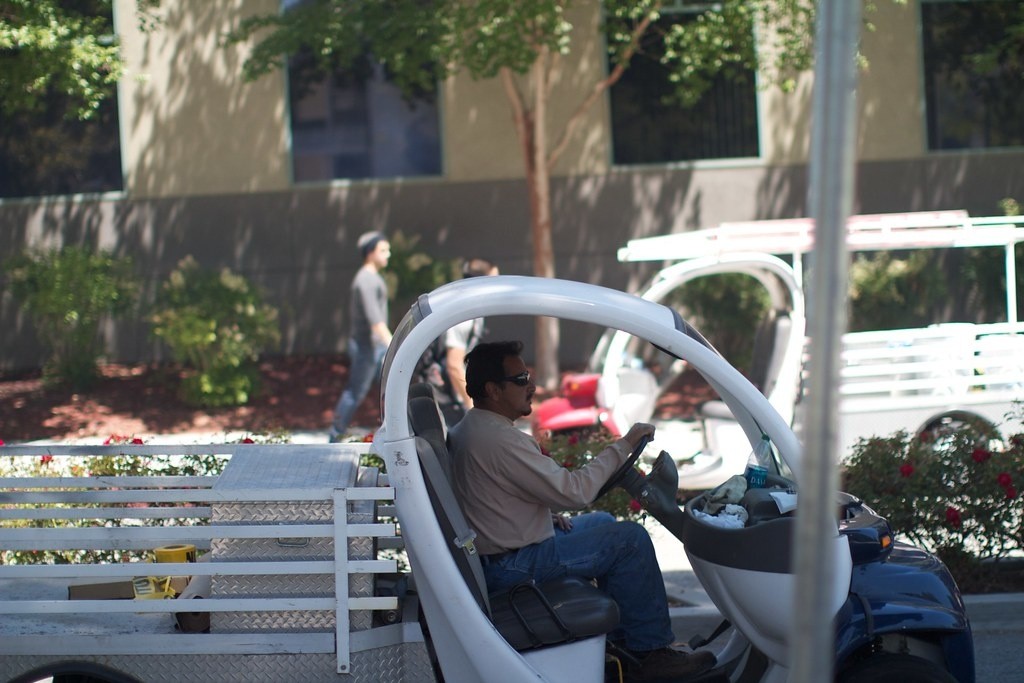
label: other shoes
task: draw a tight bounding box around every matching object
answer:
[627,646,717,683]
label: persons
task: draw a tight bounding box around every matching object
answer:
[329,230,392,443]
[414,258,499,430]
[447,340,717,683]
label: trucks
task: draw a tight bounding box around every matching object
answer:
[0,277,978,682]
[543,215,1022,487]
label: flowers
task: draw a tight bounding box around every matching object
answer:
[533,429,650,525]
[843,422,1024,597]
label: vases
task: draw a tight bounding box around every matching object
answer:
[659,581,722,644]
[949,557,1024,683]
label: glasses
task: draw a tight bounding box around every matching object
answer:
[498,370,531,386]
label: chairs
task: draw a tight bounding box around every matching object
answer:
[410,382,622,650]
